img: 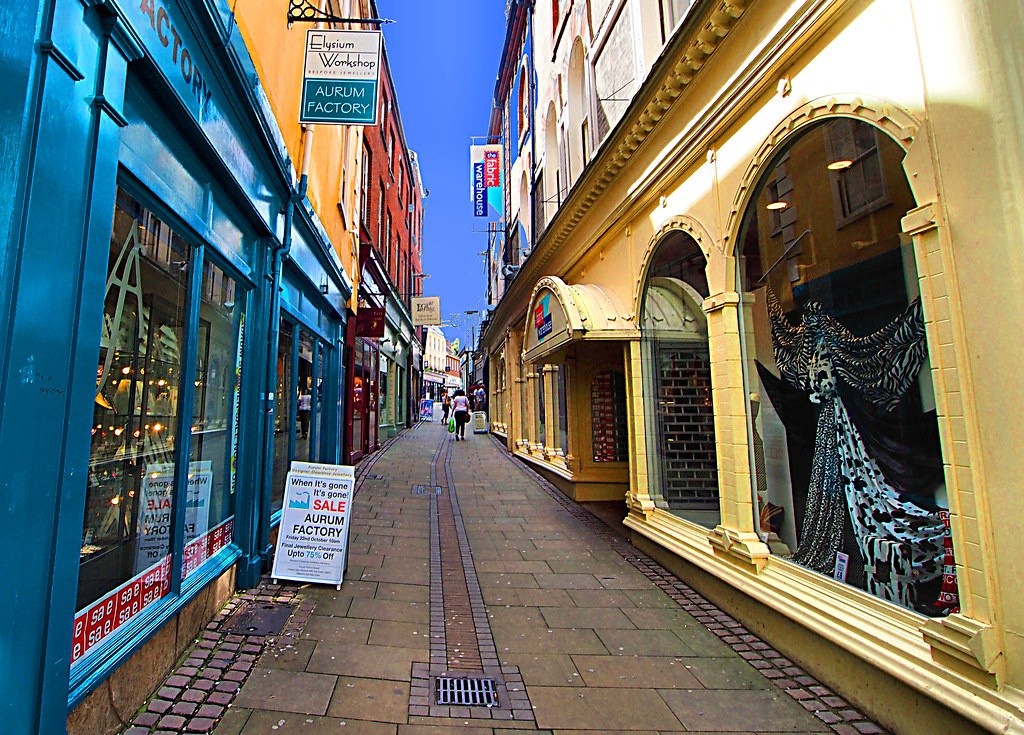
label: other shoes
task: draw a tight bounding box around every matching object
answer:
[461,437,464,440]
[441,419,443,425]
[455,435,460,441]
[445,423,449,425]
[302,433,308,440]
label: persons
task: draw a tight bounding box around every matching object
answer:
[451,390,470,440]
[440,385,486,425]
[297,389,311,440]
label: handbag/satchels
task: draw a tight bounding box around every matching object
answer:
[465,414,470,423]
[442,403,449,410]
[448,418,455,433]
